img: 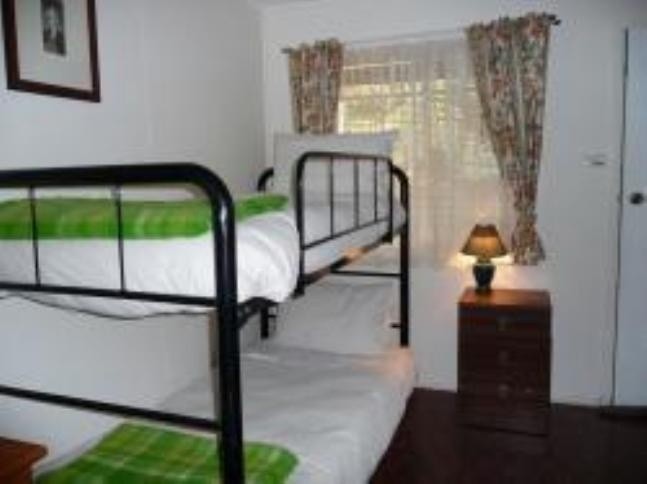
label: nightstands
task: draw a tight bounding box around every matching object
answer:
[0,438,48,484]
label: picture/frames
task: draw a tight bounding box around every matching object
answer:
[2,0,100,101]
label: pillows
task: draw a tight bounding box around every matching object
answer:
[273,129,396,197]
[270,281,393,357]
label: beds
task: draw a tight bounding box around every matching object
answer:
[1,153,415,484]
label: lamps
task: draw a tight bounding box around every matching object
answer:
[461,224,507,289]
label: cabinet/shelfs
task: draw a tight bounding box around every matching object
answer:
[457,289,552,437]
